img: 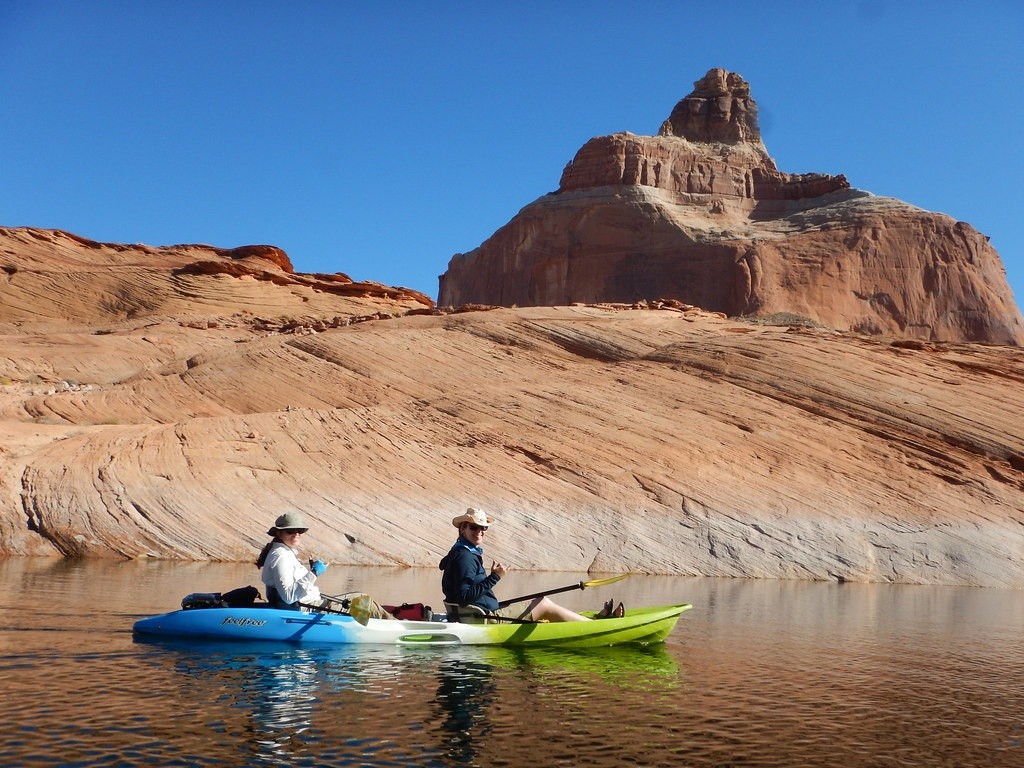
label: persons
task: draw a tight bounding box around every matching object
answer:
[438,508,625,624]
[254,513,397,620]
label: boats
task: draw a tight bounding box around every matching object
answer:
[132,603,693,646]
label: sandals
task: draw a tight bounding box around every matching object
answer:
[607,601,625,618]
[594,598,613,619]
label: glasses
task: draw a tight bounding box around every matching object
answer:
[467,524,489,532]
[285,528,305,534]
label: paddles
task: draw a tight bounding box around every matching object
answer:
[320,590,372,628]
[499,566,632,608]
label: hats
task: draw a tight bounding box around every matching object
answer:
[452,507,496,528]
[266,513,309,537]
